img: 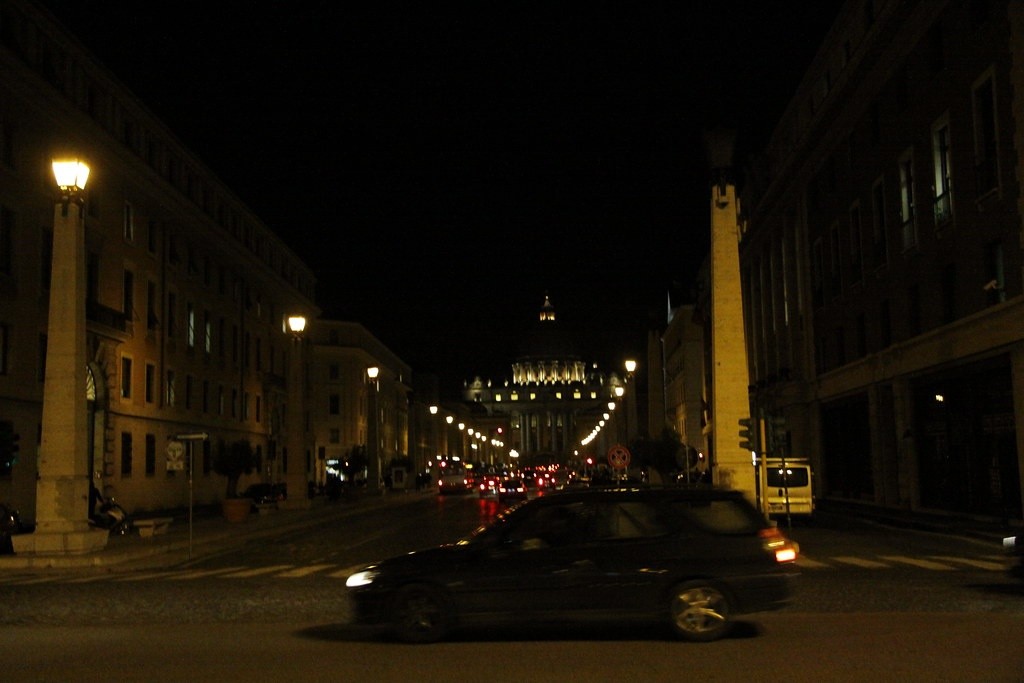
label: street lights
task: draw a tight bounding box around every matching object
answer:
[278,313,313,511]
[581,386,626,461]
[429,405,504,465]
[365,366,385,496]
[703,129,760,511]
[624,359,637,445]
[10,155,111,557]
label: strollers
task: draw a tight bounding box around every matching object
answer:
[95,499,130,536]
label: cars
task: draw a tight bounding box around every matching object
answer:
[344,489,804,642]
[437,462,591,503]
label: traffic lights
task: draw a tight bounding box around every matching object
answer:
[737,416,760,454]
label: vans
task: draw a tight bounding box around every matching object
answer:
[759,462,813,519]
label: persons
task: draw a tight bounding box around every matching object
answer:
[89,480,104,520]
